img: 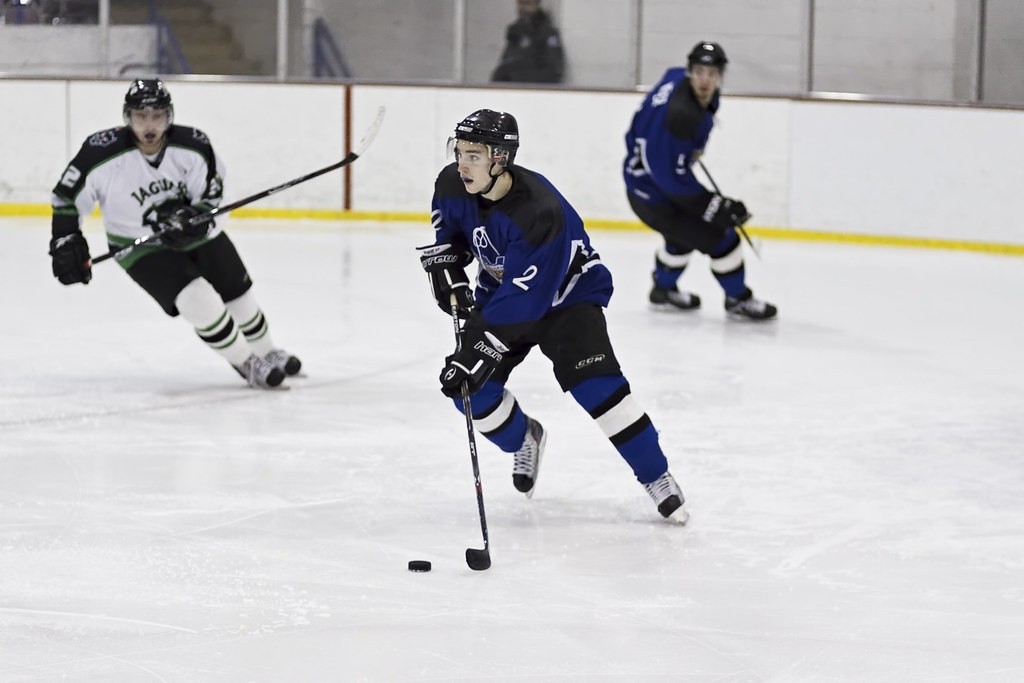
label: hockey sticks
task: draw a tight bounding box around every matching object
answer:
[443,267,494,570]
[91,105,388,266]
[695,158,764,261]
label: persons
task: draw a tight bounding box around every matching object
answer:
[623,40,778,321]
[490,0,564,84]
[49,78,301,387]
[416,109,689,529]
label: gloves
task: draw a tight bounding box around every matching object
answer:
[702,194,751,226]
[440,328,510,400]
[151,207,208,249]
[49,230,93,284]
[418,242,477,321]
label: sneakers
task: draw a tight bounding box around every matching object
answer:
[642,471,690,526]
[232,354,290,391]
[512,417,547,498]
[265,348,307,380]
[650,284,701,312]
[724,296,778,322]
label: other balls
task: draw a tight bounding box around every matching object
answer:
[408,559,431,572]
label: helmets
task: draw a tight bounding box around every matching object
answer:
[125,77,172,113]
[456,109,520,171]
[689,41,728,67]
